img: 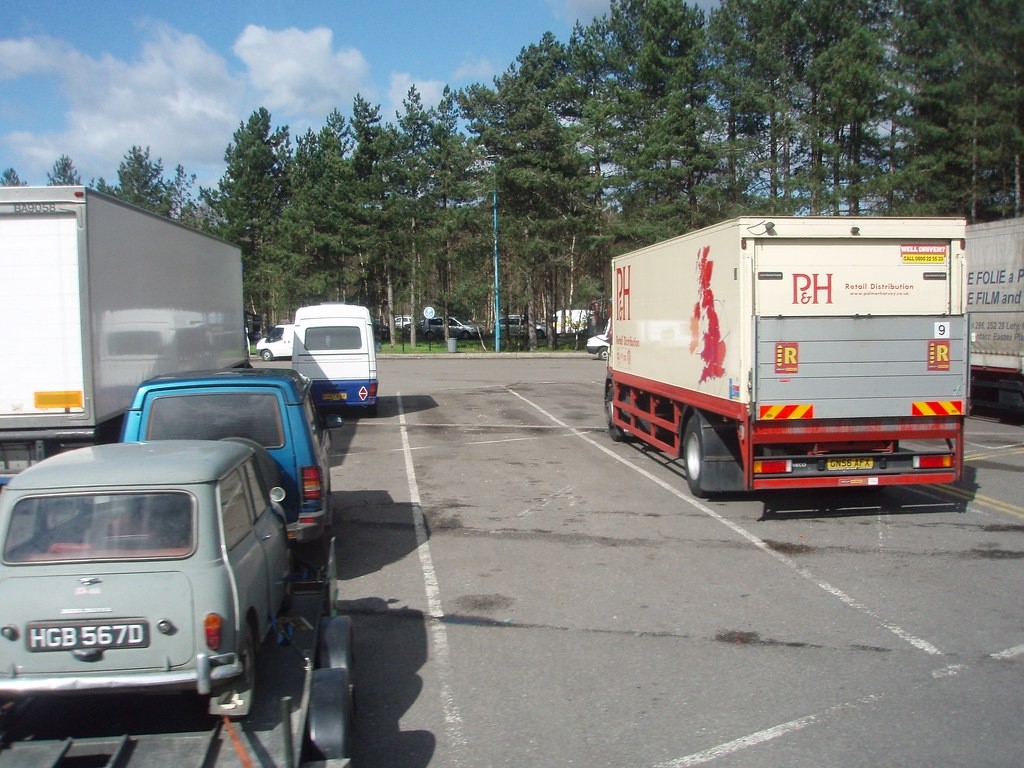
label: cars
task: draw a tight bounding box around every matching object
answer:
[419,315,484,340]
[386,316,411,329]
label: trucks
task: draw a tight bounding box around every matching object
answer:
[586,216,1024,497]
[0,184,252,475]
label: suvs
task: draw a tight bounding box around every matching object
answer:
[493,314,552,341]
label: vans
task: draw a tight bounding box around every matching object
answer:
[536,309,595,339]
[255,302,379,406]
[1,365,344,718]
